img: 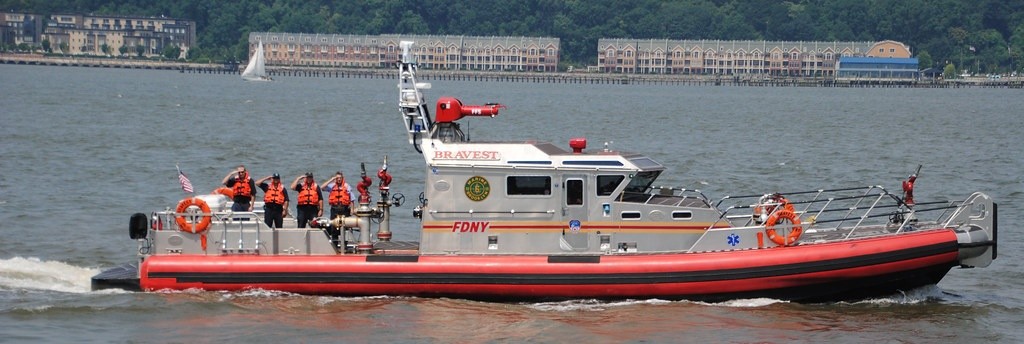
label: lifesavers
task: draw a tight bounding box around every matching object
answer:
[174,198,212,234]
[764,209,803,246]
[766,198,794,215]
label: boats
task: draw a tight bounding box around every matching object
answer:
[89,41,1000,303]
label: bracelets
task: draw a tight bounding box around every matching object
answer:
[320,209,322,210]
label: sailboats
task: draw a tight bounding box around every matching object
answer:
[240,39,272,82]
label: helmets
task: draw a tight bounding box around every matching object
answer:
[306,172,313,177]
[272,173,280,178]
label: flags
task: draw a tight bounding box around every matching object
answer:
[179,172,193,193]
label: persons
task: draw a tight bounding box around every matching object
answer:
[290,172,323,228]
[256,173,289,228]
[222,165,256,221]
[320,171,355,248]
